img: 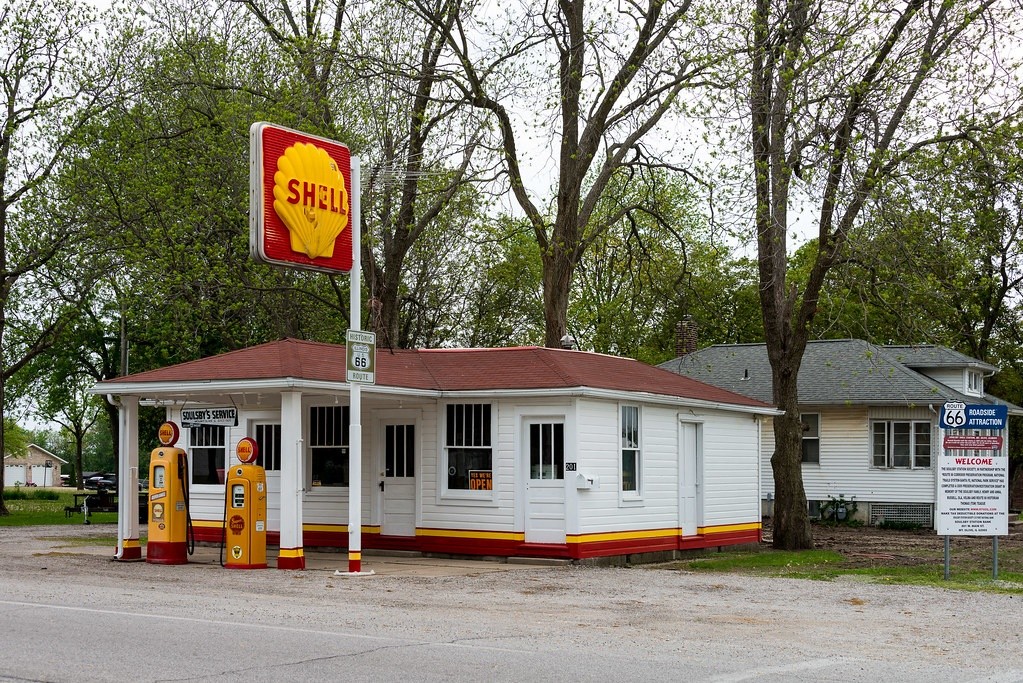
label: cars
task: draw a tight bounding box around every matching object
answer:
[60,472,150,492]
